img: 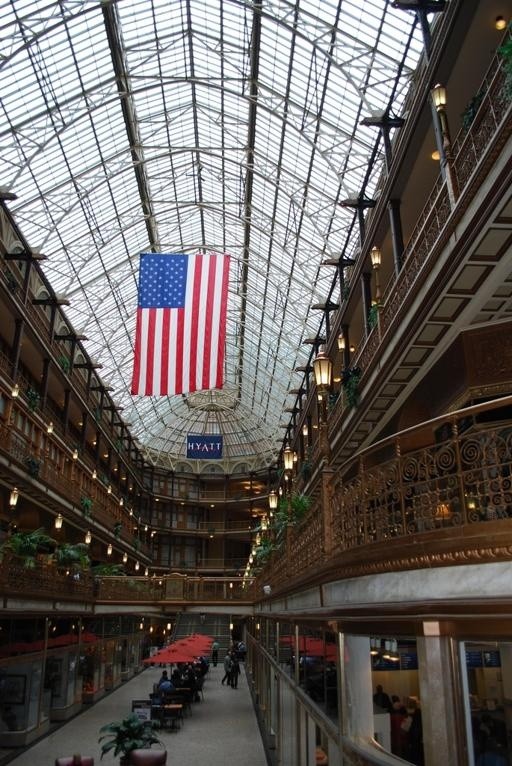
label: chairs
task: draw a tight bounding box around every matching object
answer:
[149,663,211,734]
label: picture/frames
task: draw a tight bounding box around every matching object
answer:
[0,674,27,705]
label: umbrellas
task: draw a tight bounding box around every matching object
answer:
[141,632,215,674]
[0,629,100,652]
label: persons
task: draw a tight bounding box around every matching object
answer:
[158,640,247,703]
[373,685,509,766]
[200,613,206,625]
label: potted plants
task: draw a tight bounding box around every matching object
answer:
[97,710,167,766]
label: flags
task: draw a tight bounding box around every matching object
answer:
[131,253,230,395]
